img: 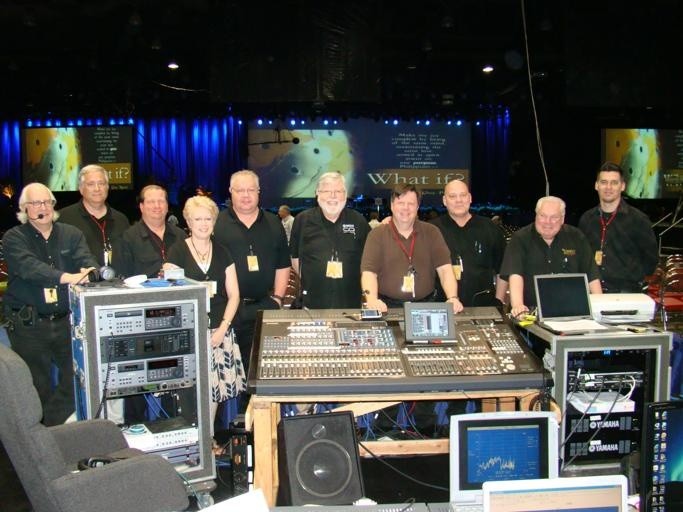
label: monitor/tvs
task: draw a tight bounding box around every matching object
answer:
[598,122,682,205]
[19,126,138,199]
[480,475,628,512]
[638,400,682,511]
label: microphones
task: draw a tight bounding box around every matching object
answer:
[165,216,178,263]
[25,207,44,220]
[99,265,115,280]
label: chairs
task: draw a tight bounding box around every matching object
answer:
[0,342,190,512]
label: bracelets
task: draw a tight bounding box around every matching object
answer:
[271,293,284,302]
[220,318,232,326]
[448,294,460,300]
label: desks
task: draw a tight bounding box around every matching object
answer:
[243,388,562,508]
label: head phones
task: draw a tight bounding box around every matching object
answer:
[78,455,115,471]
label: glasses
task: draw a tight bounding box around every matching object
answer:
[24,200,53,208]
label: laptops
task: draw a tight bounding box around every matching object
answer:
[533,272,609,333]
[448,411,559,511]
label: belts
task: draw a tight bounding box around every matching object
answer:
[12,308,71,320]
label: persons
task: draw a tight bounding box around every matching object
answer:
[491,215,507,237]
[577,162,658,293]
[56,165,130,279]
[196,185,213,199]
[430,180,508,307]
[288,171,371,309]
[166,194,241,456]
[278,205,294,244]
[213,168,292,412]
[167,210,179,227]
[500,195,602,321]
[1,183,18,242]
[121,185,188,279]
[3,181,101,427]
[360,183,464,315]
[368,211,380,229]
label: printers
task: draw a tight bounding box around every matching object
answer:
[588,292,657,323]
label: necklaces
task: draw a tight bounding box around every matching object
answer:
[191,236,211,272]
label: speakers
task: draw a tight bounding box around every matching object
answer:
[277,410,365,507]
